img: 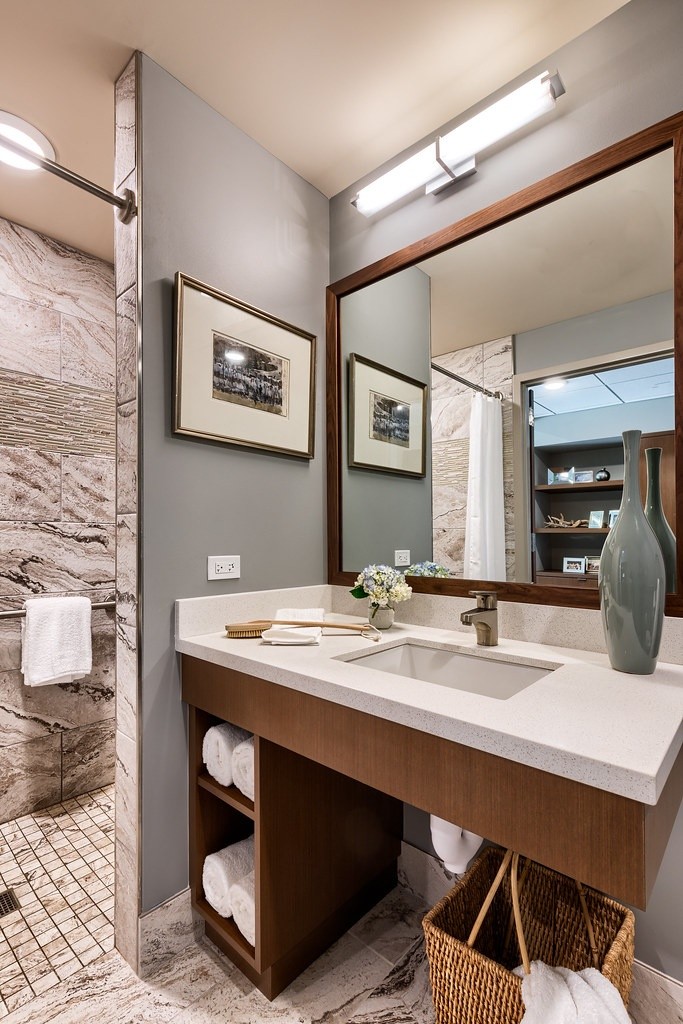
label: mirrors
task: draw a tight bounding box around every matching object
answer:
[326,109,683,618]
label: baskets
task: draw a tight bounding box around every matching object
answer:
[422,846,633,1024]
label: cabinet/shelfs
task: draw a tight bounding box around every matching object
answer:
[534,480,624,588]
[181,653,404,1003]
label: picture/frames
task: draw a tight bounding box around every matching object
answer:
[563,557,585,573]
[588,511,604,528]
[172,270,317,460]
[574,471,594,483]
[584,556,601,573]
[348,352,428,478]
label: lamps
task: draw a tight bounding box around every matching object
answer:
[350,67,567,219]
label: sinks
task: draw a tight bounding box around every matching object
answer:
[330,637,565,700]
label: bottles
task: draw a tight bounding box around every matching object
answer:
[597,430,665,674]
[644,447,676,594]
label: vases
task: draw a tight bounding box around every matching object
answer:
[367,598,394,630]
[643,447,676,594]
[597,430,665,675]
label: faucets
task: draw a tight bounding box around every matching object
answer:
[460,589,499,647]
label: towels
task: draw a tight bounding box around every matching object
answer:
[261,608,325,646]
[202,833,255,919]
[230,735,254,804]
[202,723,252,787]
[228,870,255,947]
[511,960,632,1024]
[20,596,92,687]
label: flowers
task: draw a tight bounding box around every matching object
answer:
[404,560,456,579]
[348,563,412,619]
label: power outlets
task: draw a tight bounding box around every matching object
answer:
[394,550,410,567]
[207,555,240,580]
